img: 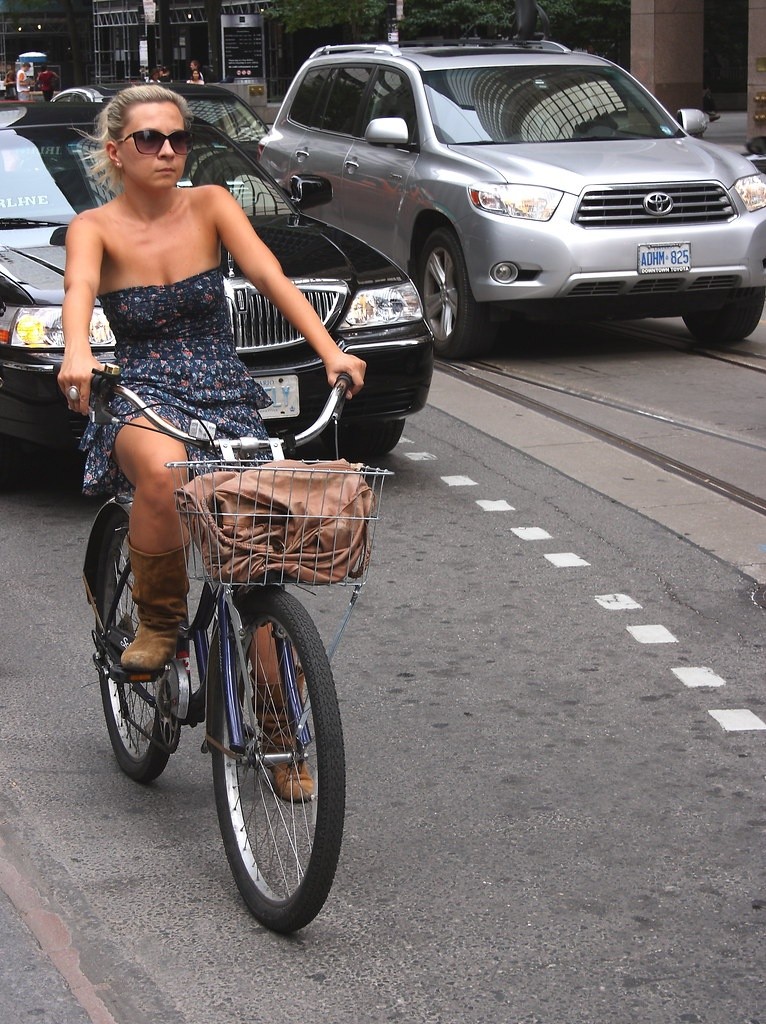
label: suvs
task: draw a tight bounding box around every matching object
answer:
[254,38,766,364]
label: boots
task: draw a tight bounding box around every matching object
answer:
[249,665,315,803]
[121,533,192,672]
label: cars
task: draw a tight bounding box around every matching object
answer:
[1,86,438,492]
[51,80,272,166]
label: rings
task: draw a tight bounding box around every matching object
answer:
[67,386,80,403]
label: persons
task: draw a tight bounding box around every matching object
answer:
[0,61,60,102]
[57,87,370,805]
[139,59,206,86]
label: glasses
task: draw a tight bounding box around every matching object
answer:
[117,129,194,155]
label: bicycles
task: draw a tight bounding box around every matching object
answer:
[47,358,395,934]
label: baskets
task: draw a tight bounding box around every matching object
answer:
[165,458,396,587]
